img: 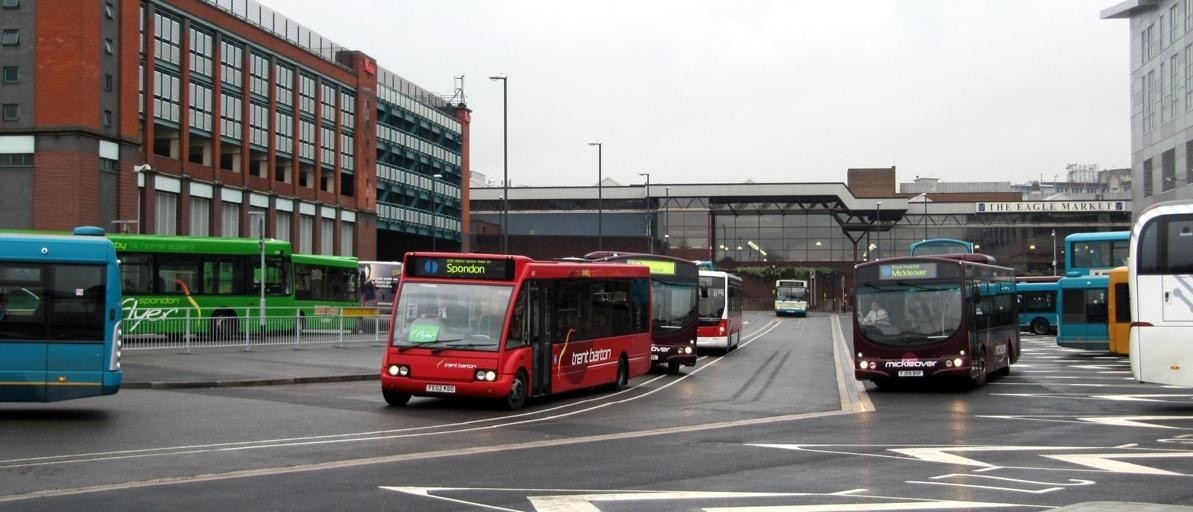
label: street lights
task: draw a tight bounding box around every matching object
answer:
[639,173,650,253]
[488,75,508,253]
[588,142,601,250]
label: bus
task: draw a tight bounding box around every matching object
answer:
[771,279,809,317]
[0,226,123,403]
[356,260,402,334]
[291,253,363,334]
[0,229,297,340]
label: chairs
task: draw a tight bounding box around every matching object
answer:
[417,303,501,336]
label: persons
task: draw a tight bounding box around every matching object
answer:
[124,278,135,293]
[862,300,891,326]
[422,293,454,323]
